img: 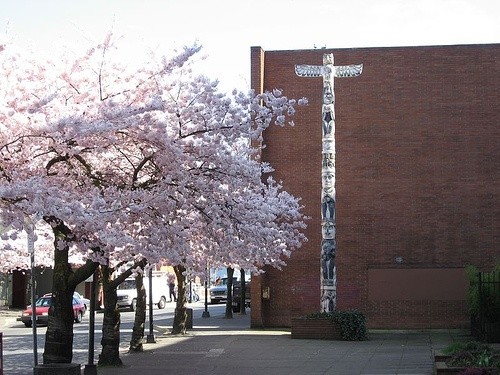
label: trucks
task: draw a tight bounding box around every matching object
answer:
[114,275,170,312]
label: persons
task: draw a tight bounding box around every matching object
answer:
[216,276,222,285]
[167,271,177,301]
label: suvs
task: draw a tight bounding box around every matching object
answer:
[210,276,237,304]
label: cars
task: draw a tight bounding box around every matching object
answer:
[22,292,88,328]
